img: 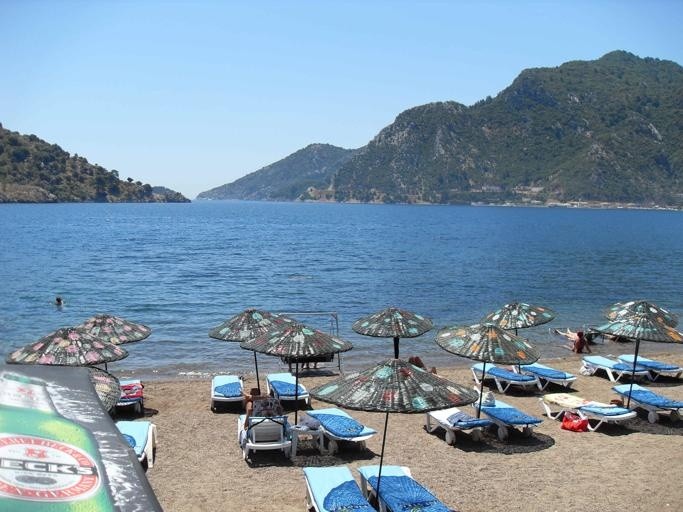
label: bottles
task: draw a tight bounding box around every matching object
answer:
[0,371,120,512]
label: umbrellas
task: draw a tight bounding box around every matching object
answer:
[435,322,541,418]
[483,302,558,374]
[209,309,296,391]
[309,359,479,505]
[595,312,683,409]
[240,322,353,425]
[5,326,129,366]
[75,314,152,370]
[352,308,434,359]
[86,368,119,411]
[602,300,680,322]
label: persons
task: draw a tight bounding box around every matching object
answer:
[243,388,260,429]
[562,331,591,354]
[409,356,436,374]
[556,328,578,340]
[55,298,63,306]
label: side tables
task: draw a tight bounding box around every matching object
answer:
[289,425,325,456]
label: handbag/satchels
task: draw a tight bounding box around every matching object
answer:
[560,410,590,432]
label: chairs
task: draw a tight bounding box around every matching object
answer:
[471,363,577,394]
[611,384,683,424]
[238,414,291,460]
[579,355,683,382]
[543,393,637,432]
[266,373,309,404]
[211,376,244,408]
[306,409,377,452]
[116,380,144,412]
[302,466,454,512]
[427,385,542,445]
[115,421,157,467]
